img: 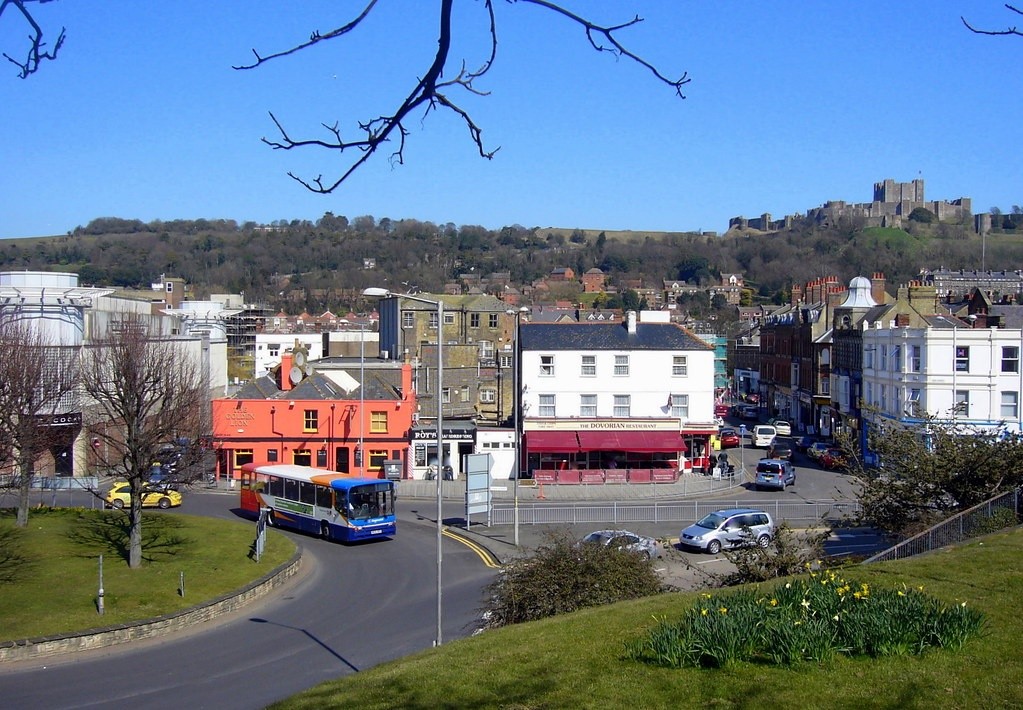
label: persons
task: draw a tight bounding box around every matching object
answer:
[708,451,718,475]
[717,448,729,472]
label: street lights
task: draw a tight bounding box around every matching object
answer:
[935,316,958,507]
[505,306,529,547]
[361,287,447,648]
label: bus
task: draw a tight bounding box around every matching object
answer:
[240,463,398,545]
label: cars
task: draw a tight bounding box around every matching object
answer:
[818,447,848,471]
[153,453,178,471]
[139,462,180,492]
[163,448,182,460]
[742,407,757,419]
[766,418,783,427]
[733,404,747,417]
[806,442,833,459]
[107,481,182,509]
[719,429,740,449]
[570,529,657,567]
[772,421,791,437]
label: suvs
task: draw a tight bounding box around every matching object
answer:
[795,436,818,452]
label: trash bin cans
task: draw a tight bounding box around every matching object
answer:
[383,460,402,483]
[728,465,735,479]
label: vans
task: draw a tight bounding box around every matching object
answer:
[716,405,728,419]
[755,459,797,491]
[751,425,777,447]
[679,507,775,554]
[767,442,794,464]
